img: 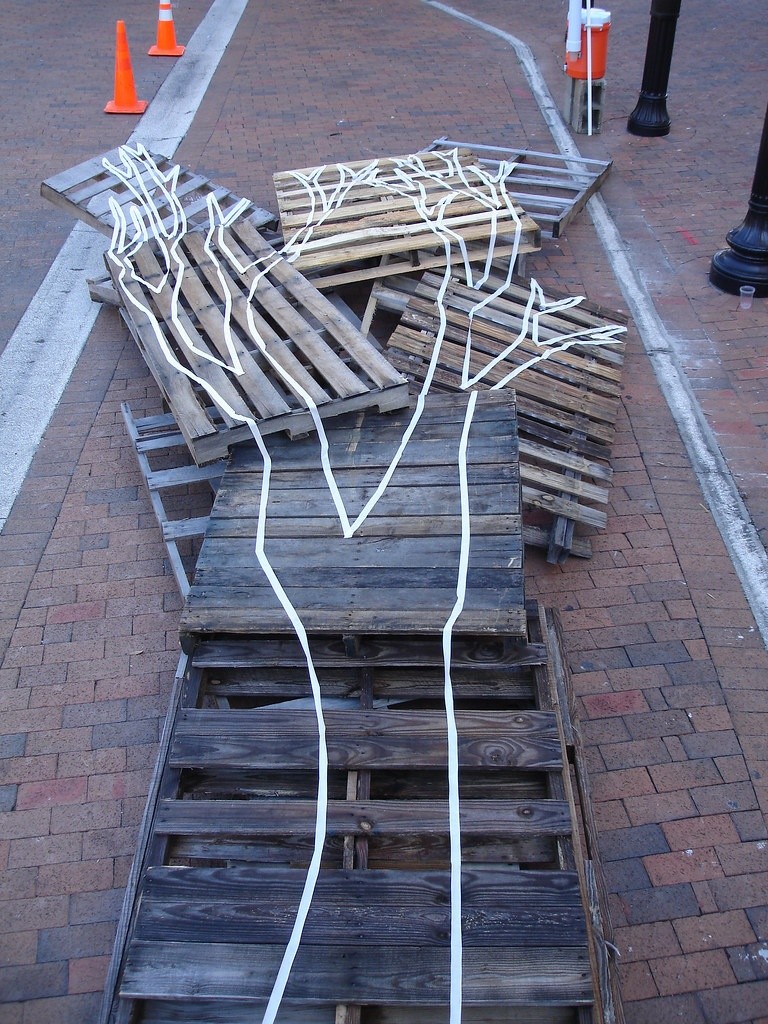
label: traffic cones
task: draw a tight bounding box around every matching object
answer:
[148,0,185,56]
[104,21,149,114]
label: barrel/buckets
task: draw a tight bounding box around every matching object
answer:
[564,8,612,80]
